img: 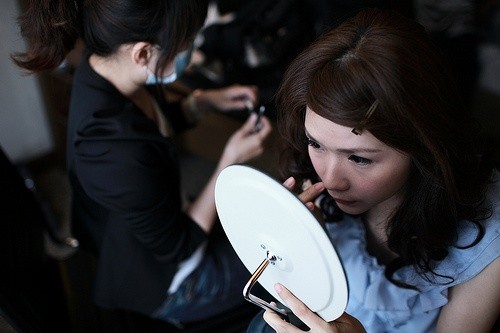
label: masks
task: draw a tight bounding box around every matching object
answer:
[139,38,196,85]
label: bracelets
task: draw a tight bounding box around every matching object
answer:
[189,88,202,118]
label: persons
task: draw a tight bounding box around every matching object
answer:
[11,0,273,327]
[263,10,500,332]
